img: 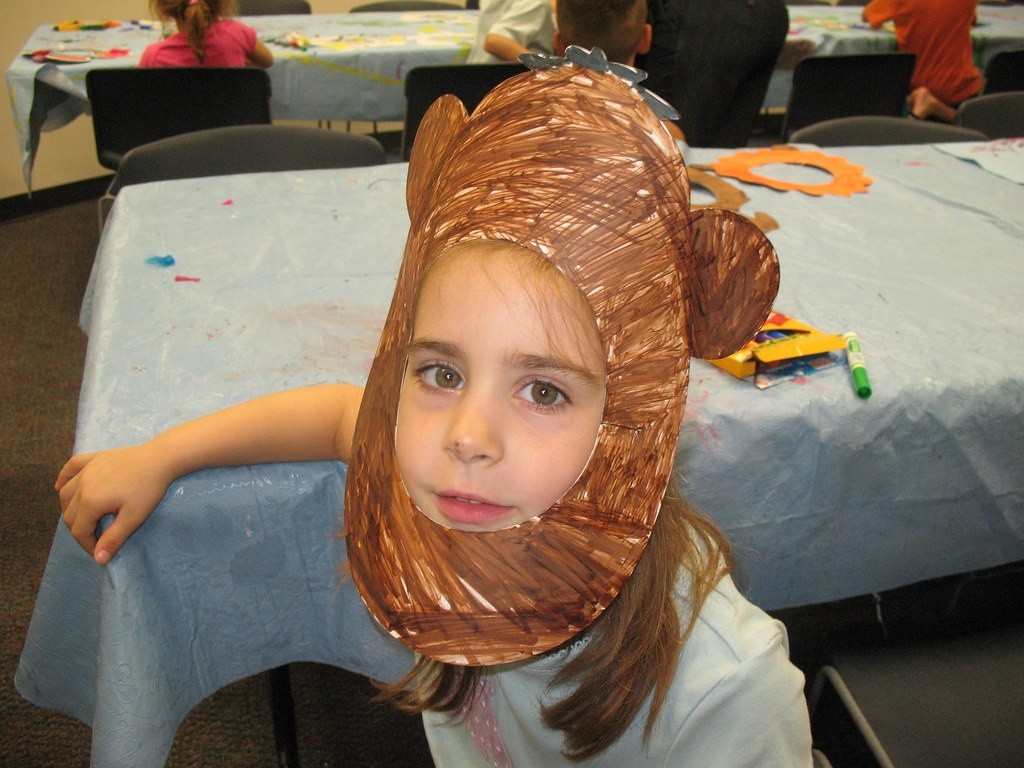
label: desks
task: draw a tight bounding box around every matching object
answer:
[11,0,1024,191]
[13,140,1024,768]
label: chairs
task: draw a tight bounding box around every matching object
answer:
[399,54,547,162]
[96,122,390,223]
[955,90,1024,140]
[83,59,272,169]
[234,0,331,131]
[983,47,1024,94]
[786,116,988,149]
[779,50,925,143]
[344,0,465,133]
[806,612,1024,768]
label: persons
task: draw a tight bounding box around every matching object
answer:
[138,0,273,68]
[53,66,812,763]
[484,0,685,141]
[861,0,982,125]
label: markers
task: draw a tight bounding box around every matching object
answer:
[843,333,872,399]
[292,33,308,44]
[131,20,160,29]
[768,331,786,338]
[286,35,298,47]
[756,334,775,342]
[294,37,307,51]
[745,341,778,367]
